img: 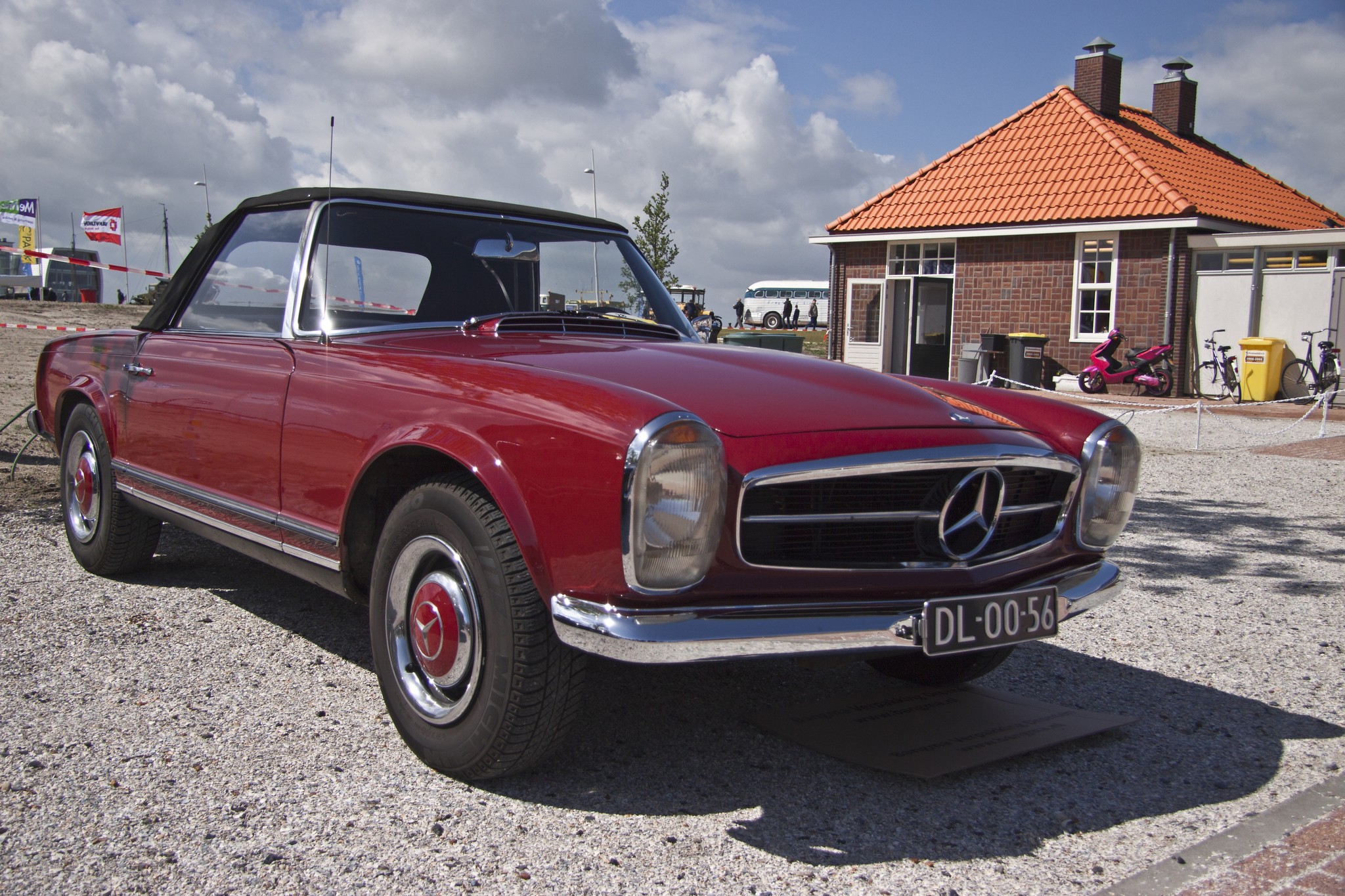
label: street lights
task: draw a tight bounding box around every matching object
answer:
[583,148,609,310]
[193,163,212,227]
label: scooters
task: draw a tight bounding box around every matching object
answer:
[1077,326,1175,397]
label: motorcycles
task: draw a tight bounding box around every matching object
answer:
[711,315,723,329]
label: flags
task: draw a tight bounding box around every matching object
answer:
[1,199,38,229]
[80,207,122,245]
[19,225,37,265]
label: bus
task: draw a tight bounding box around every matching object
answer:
[742,280,829,329]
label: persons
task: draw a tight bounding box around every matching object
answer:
[781,298,792,329]
[805,299,818,331]
[684,299,702,323]
[61,291,69,302]
[117,289,125,305]
[30,287,41,301]
[733,298,744,328]
[48,287,57,302]
[792,305,800,330]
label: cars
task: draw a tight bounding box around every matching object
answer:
[22,187,1139,781]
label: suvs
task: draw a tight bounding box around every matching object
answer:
[135,282,167,302]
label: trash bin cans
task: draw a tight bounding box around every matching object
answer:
[957,358,979,384]
[79,288,97,303]
[1239,336,1287,403]
[1007,331,1051,390]
[721,331,805,354]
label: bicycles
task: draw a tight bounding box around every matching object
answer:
[1280,328,1341,409]
[1192,329,1242,404]
